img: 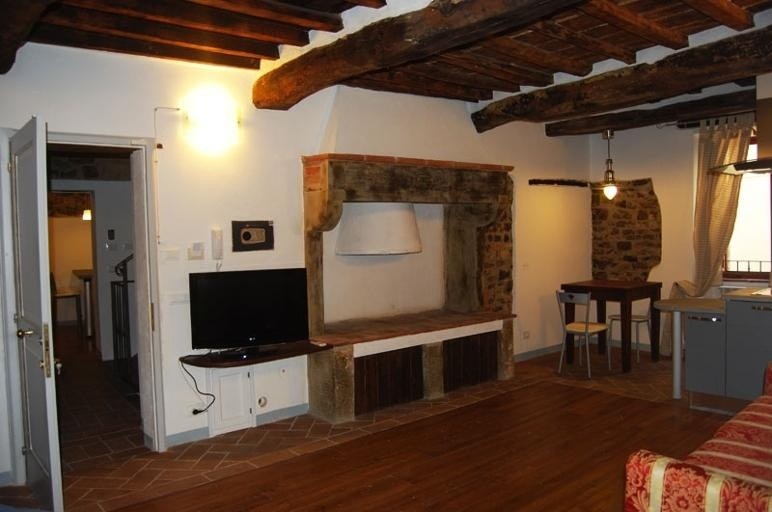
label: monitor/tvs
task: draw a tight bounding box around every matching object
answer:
[188,267,310,363]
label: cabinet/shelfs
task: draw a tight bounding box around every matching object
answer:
[312,312,515,426]
[654,298,724,415]
[725,286,772,419]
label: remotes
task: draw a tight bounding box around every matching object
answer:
[310,340,327,348]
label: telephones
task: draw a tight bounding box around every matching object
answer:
[212,229,223,260]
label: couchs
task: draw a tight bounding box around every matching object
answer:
[625,364,771,512]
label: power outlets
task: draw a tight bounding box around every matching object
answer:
[189,404,205,417]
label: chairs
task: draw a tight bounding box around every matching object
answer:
[608,300,652,365]
[555,290,612,379]
[50,272,82,345]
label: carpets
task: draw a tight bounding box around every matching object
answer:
[112,377,750,512]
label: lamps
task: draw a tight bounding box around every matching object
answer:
[601,129,618,202]
[82,209,92,223]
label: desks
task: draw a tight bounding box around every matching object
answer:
[560,280,662,372]
[73,269,95,338]
